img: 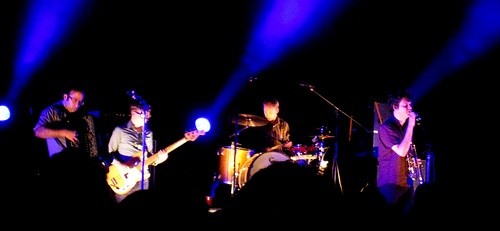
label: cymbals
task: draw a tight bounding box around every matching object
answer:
[231,114,268,127]
[313,135,335,139]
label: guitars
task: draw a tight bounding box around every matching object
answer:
[106,130,205,194]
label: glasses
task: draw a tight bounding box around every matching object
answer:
[67,95,85,106]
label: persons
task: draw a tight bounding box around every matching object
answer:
[105,99,169,204]
[263,99,293,157]
[32,86,86,158]
[376,89,422,215]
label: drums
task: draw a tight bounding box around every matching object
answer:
[290,154,317,164]
[219,146,253,184]
[308,145,321,154]
[290,144,308,155]
[239,152,290,188]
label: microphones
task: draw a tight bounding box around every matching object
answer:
[128,91,142,101]
[411,112,419,122]
[299,83,315,88]
